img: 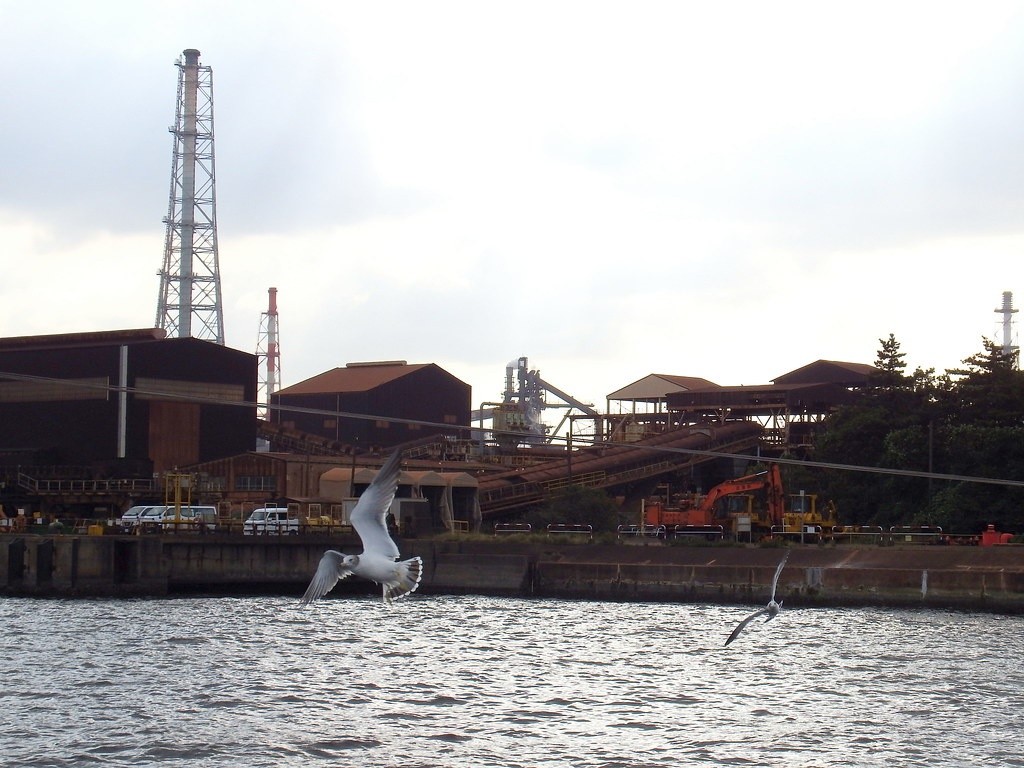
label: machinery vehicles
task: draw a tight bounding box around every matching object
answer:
[782,493,862,542]
[640,463,786,543]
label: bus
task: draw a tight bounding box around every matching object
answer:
[139,506,218,531]
[122,506,154,533]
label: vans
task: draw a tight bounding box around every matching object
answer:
[243,507,300,536]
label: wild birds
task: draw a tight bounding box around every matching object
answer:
[294,446,423,610]
[724,548,790,647]
